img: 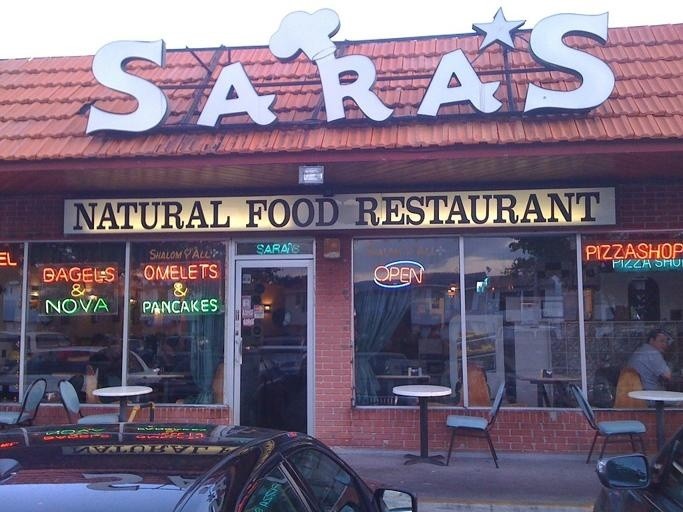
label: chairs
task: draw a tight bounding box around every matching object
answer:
[457,363,491,407]
[56,379,119,424]
[566,383,647,464]
[443,382,506,469]
[612,369,647,408]
[1,377,47,427]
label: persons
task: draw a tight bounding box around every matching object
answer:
[628,329,672,406]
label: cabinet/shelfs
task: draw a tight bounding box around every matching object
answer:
[550,323,683,392]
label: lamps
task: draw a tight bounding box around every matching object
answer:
[298,165,325,187]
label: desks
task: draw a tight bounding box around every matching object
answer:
[626,388,682,455]
[374,375,431,385]
[520,372,580,406]
[391,385,453,465]
[129,371,186,403]
[91,385,153,421]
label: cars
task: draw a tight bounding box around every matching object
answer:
[0,418,419,512]
[588,424,683,512]
